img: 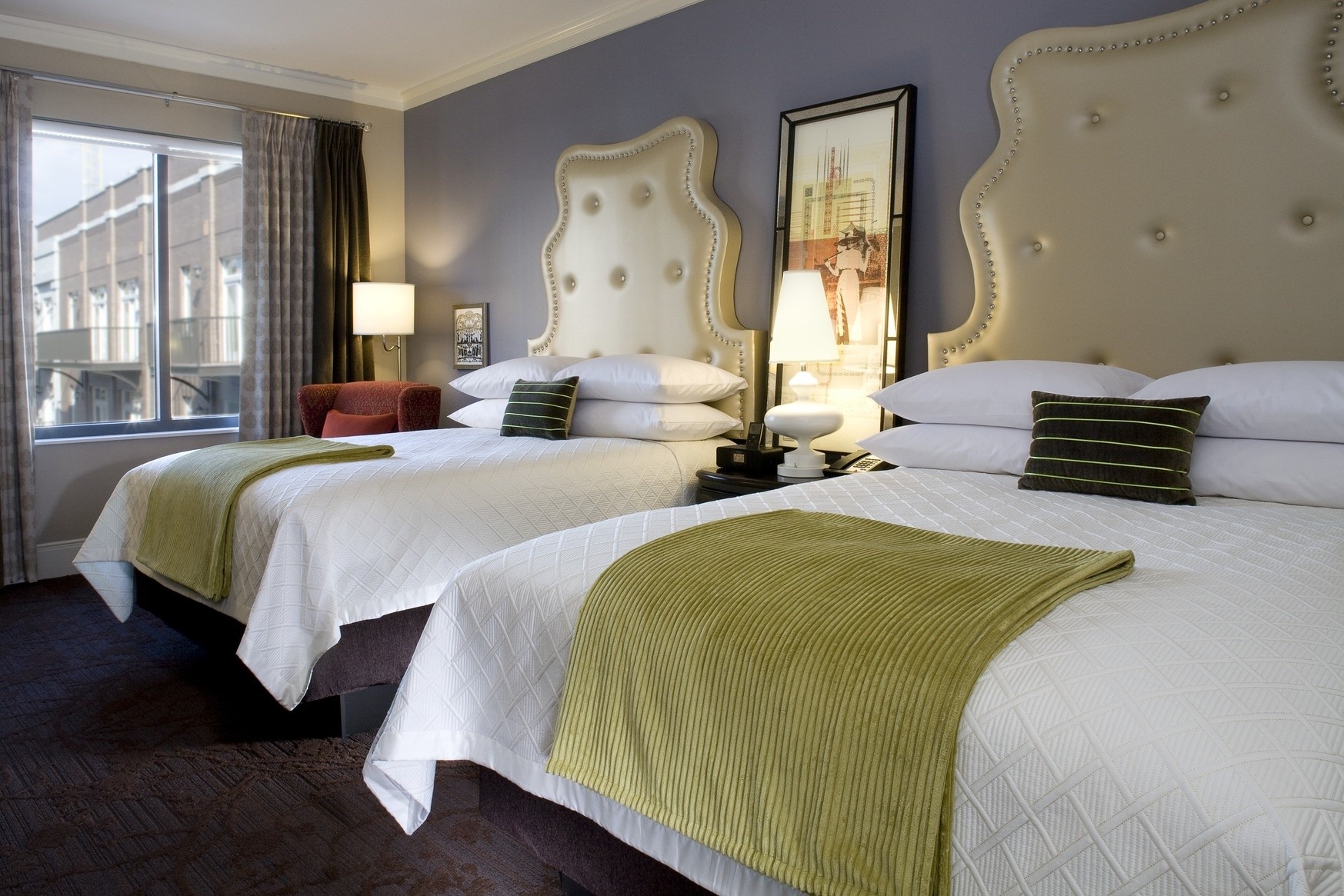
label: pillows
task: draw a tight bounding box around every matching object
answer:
[446,399,509,430]
[854,423,1035,478]
[499,376,580,441]
[1125,361,1344,445]
[1017,391,1211,508]
[449,356,588,399]
[1184,437,1344,510]
[866,360,1157,433]
[569,397,743,442]
[321,410,398,438]
[552,353,748,404]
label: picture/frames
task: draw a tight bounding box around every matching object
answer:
[452,303,489,370]
[755,83,919,461]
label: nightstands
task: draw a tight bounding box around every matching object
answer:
[695,466,831,505]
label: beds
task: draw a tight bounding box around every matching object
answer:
[70,113,767,738]
[360,0,1344,896]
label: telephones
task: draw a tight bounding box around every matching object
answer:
[822,449,888,477]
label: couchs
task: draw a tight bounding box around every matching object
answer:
[297,380,441,440]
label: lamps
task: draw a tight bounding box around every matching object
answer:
[762,268,843,479]
[353,282,415,381]
